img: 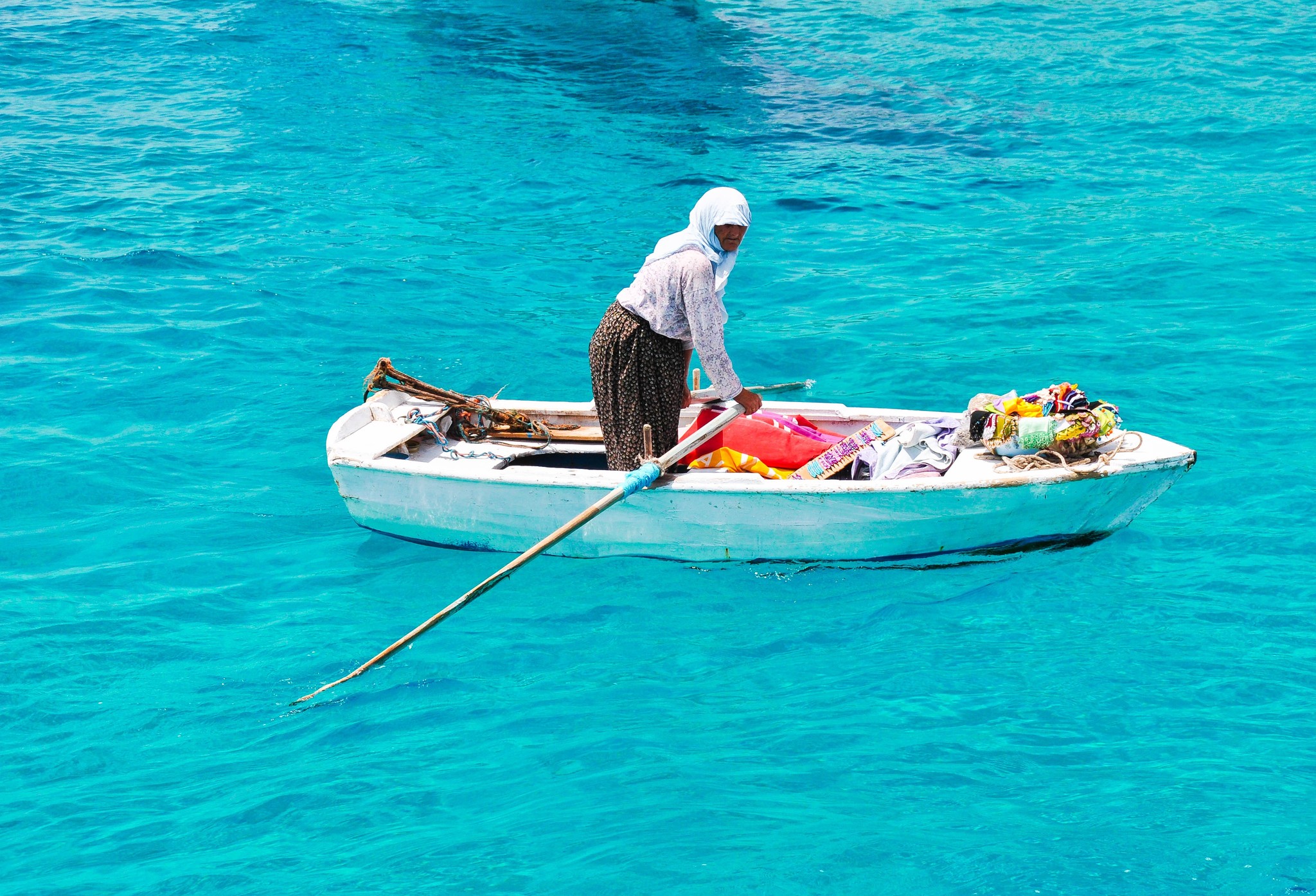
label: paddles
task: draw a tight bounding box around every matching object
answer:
[688,380,811,402]
[289,398,753,707]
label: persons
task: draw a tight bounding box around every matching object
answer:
[588,186,762,470]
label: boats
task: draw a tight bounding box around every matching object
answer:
[326,386,1197,564]
